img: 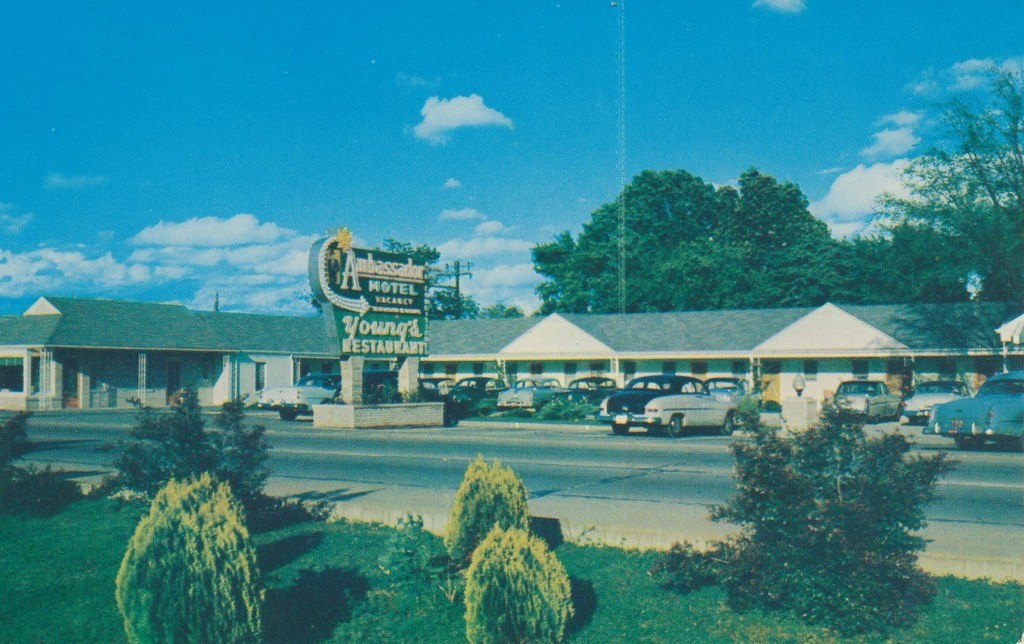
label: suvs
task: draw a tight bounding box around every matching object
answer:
[333,369,399,405]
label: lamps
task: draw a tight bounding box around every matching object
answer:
[792,367,807,398]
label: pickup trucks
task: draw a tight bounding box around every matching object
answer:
[257,372,340,421]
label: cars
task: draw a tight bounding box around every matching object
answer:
[833,380,903,423]
[554,377,621,418]
[600,375,735,439]
[417,377,506,416]
[897,381,973,421]
[499,378,563,413]
[701,377,744,397]
[922,371,1024,450]
